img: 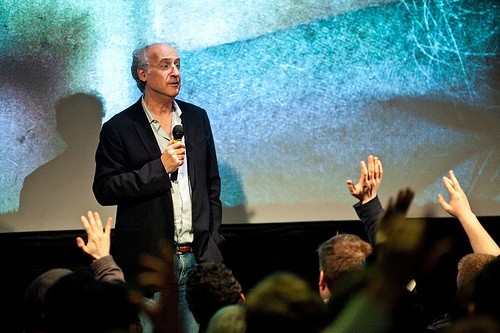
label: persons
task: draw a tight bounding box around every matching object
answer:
[0,156,500,333]
[92,45,226,333]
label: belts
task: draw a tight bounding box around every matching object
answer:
[176,246,193,254]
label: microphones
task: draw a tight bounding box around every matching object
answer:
[171,125,184,182]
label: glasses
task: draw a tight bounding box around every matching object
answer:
[150,63,180,71]
